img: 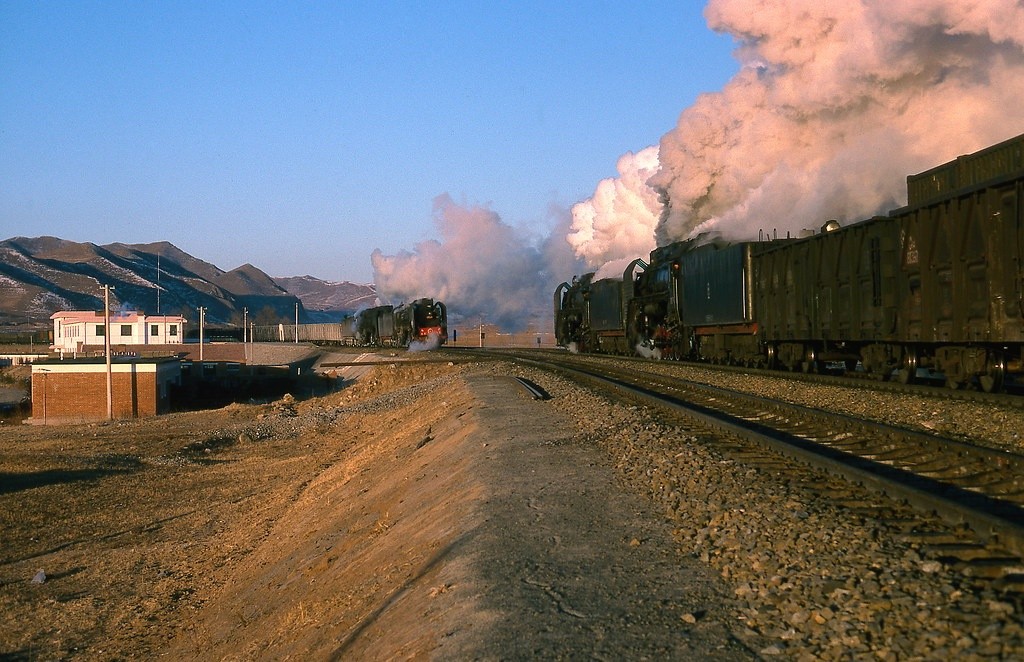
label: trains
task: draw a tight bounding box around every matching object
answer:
[187,295,448,352]
[547,131,1024,394]
